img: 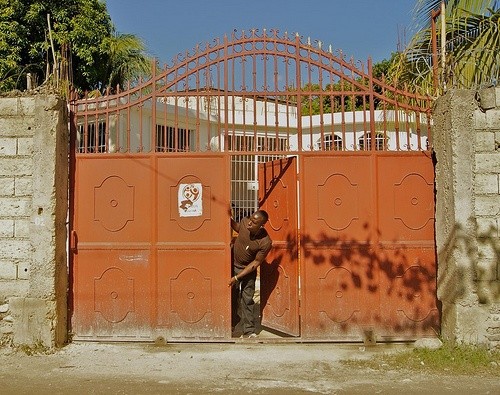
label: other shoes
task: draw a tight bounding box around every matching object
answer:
[244,332,259,339]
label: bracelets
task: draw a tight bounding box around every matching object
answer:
[233,275,239,282]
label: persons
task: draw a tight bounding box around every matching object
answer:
[227,209,272,341]
